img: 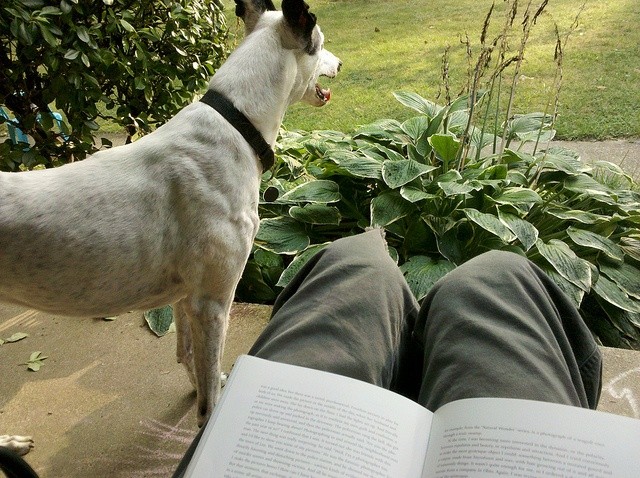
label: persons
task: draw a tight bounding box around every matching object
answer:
[169,226,603,478]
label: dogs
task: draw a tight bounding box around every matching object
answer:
[1,0,343,456]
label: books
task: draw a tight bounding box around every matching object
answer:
[182,353,639,478]
